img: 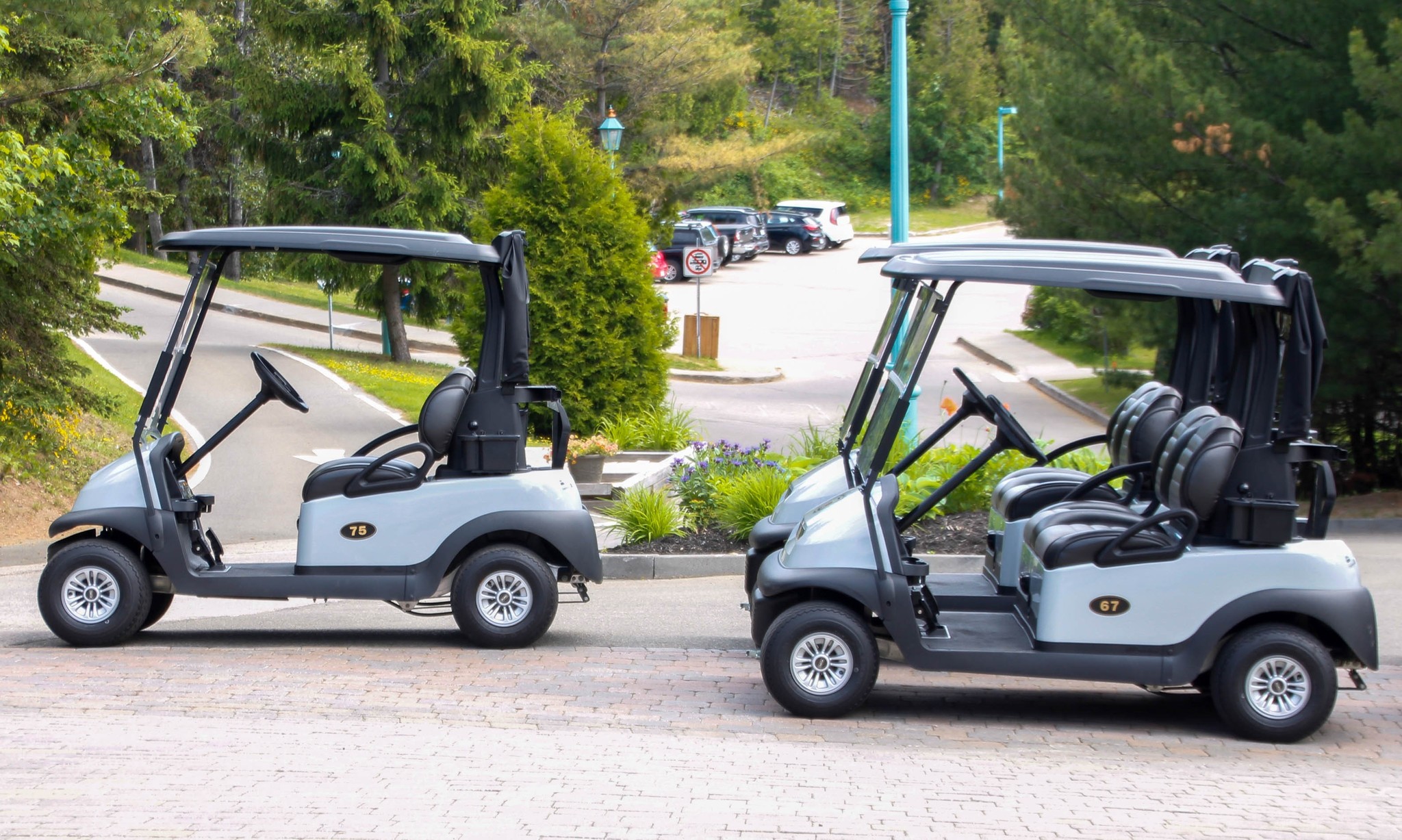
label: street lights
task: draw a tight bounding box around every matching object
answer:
[598,105,625,201]
[997,106,1018,203]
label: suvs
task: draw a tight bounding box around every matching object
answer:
[769,200,854,248]
[651,219,730,284]
[760,210,827,257]
[670,206,770,262]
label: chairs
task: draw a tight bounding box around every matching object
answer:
[302,365,477,503]
[990,380,1244,572]
